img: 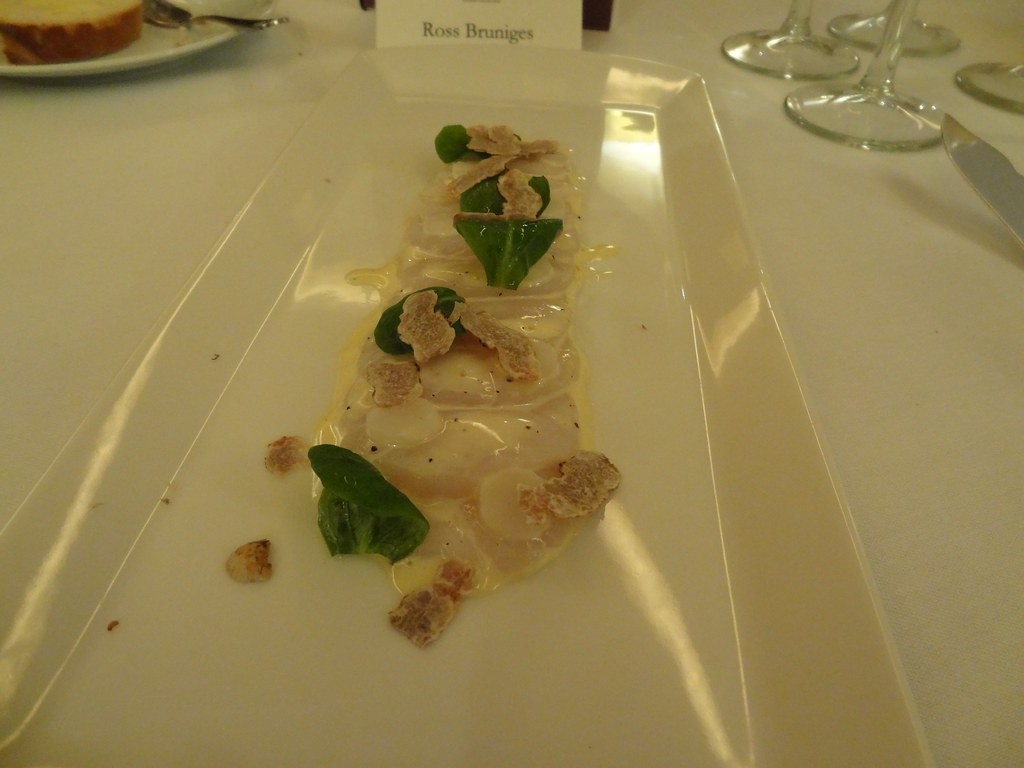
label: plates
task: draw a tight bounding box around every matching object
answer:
[0,0,277,77]
[0,47,937,768]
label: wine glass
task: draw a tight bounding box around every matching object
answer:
[721,0,1024,156]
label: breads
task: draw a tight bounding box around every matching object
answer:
[0,0,143,65]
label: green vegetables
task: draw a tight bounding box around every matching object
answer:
[374,287,468,355]
[307,443,430,565]
[435,125,562,289]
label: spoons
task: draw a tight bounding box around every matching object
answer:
[142,0,289,29]
